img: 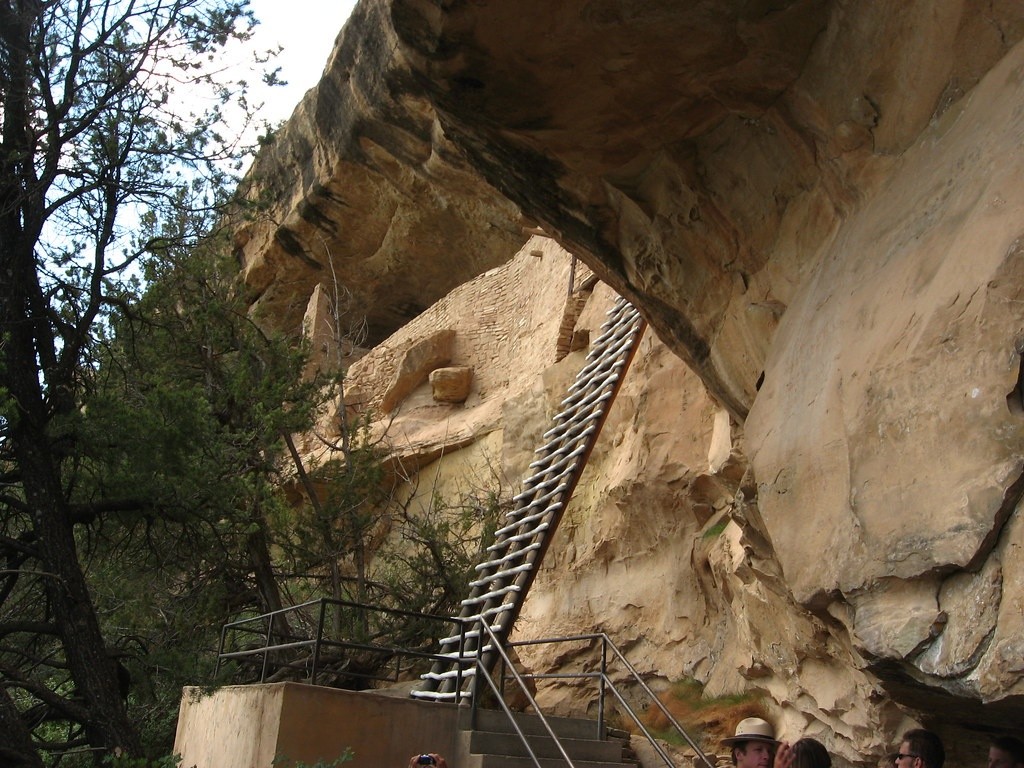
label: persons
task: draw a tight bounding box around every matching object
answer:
[408,753,447,768]
[719,717,789,768]
[878,752,899,768]
[987,736,1024,768]
[774,737,832,768]
[895,729,945,768]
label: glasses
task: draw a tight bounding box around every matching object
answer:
[896,753,921,760]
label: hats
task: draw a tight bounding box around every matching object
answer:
[720,717,782,751]
